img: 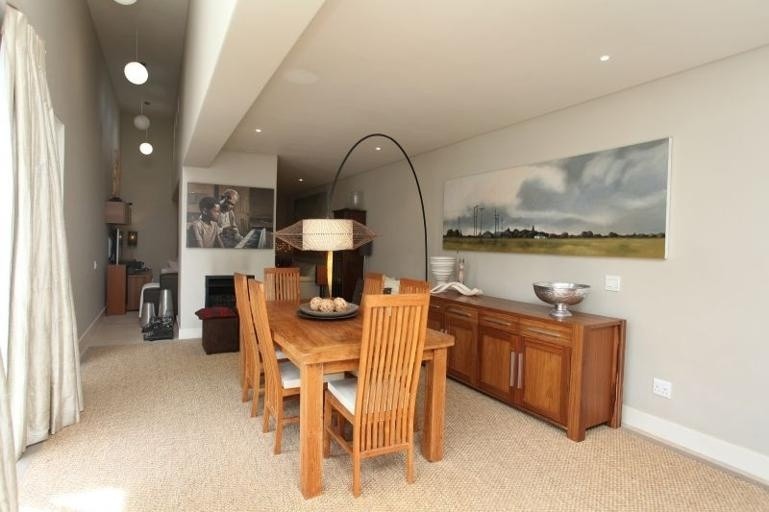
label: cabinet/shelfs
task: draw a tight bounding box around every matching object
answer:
[107,201,132,225]
[205,274,254,307]
[427,295,477,392]
[477,312,622,444]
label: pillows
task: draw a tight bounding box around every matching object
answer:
[194,307,237,319]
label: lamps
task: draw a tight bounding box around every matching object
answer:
[275,133,430,281]
[125,30,155,157]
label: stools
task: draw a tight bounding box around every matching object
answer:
[196,305,239,357]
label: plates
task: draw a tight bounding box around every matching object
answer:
[296,301,359,320]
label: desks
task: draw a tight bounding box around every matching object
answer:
[158,274,176,315]
[105,259,152,315]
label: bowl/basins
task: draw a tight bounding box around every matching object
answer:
[532,280,592,306]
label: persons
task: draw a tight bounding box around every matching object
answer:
[199,190,246,249]
[189,195,220,249]
